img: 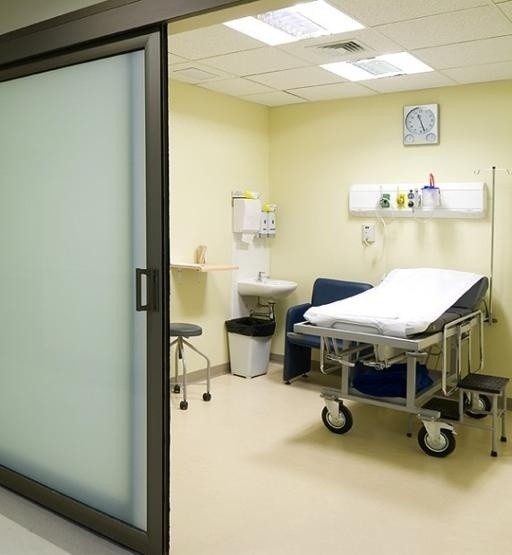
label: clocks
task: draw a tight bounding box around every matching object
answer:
[402,104,438,144]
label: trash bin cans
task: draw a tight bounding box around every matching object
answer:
[225,317,276,379]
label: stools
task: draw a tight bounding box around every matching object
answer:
[456,371,510,456]
[170,323,212,409]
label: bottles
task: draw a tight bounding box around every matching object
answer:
[380,194,404,208]
[406,189,420,208]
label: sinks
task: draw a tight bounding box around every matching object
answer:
[238,278,299,300]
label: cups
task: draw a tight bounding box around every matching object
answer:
[422,187,440,209]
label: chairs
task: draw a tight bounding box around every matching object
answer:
[284,278,373,384]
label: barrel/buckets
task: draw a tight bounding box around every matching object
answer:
[420,171,440,208]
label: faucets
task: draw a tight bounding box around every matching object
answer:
[257,271,271,281]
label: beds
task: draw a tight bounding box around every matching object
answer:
[292,266,490,456]
